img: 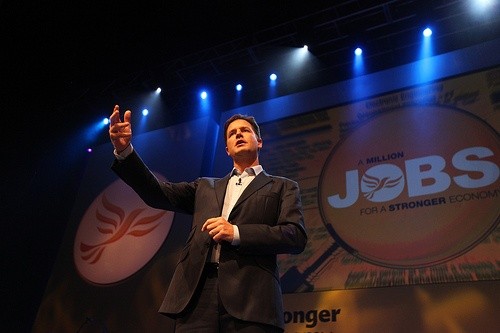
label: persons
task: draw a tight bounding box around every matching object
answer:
[108,103,307,333]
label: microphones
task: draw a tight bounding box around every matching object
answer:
[235,178,242,185]
